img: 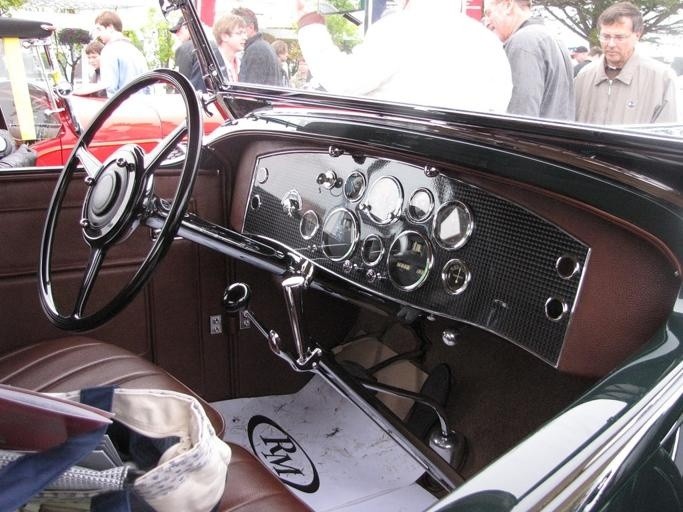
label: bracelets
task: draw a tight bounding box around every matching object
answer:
[292,12,326,32]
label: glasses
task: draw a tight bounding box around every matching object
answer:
[597,33,627,43]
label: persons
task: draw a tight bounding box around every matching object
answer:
[671,58,683,123]
[484,0,576,121]
[570,46,603,78]
[287,0,514,113]
[575,1,678,125]
[1,129,37,168]
[73,11,152,98]
[263,34,327,92]
[170,6,283,94]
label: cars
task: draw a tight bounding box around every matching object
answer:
[0,12,228,172]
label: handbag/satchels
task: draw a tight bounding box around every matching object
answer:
[1,388,232,512]
